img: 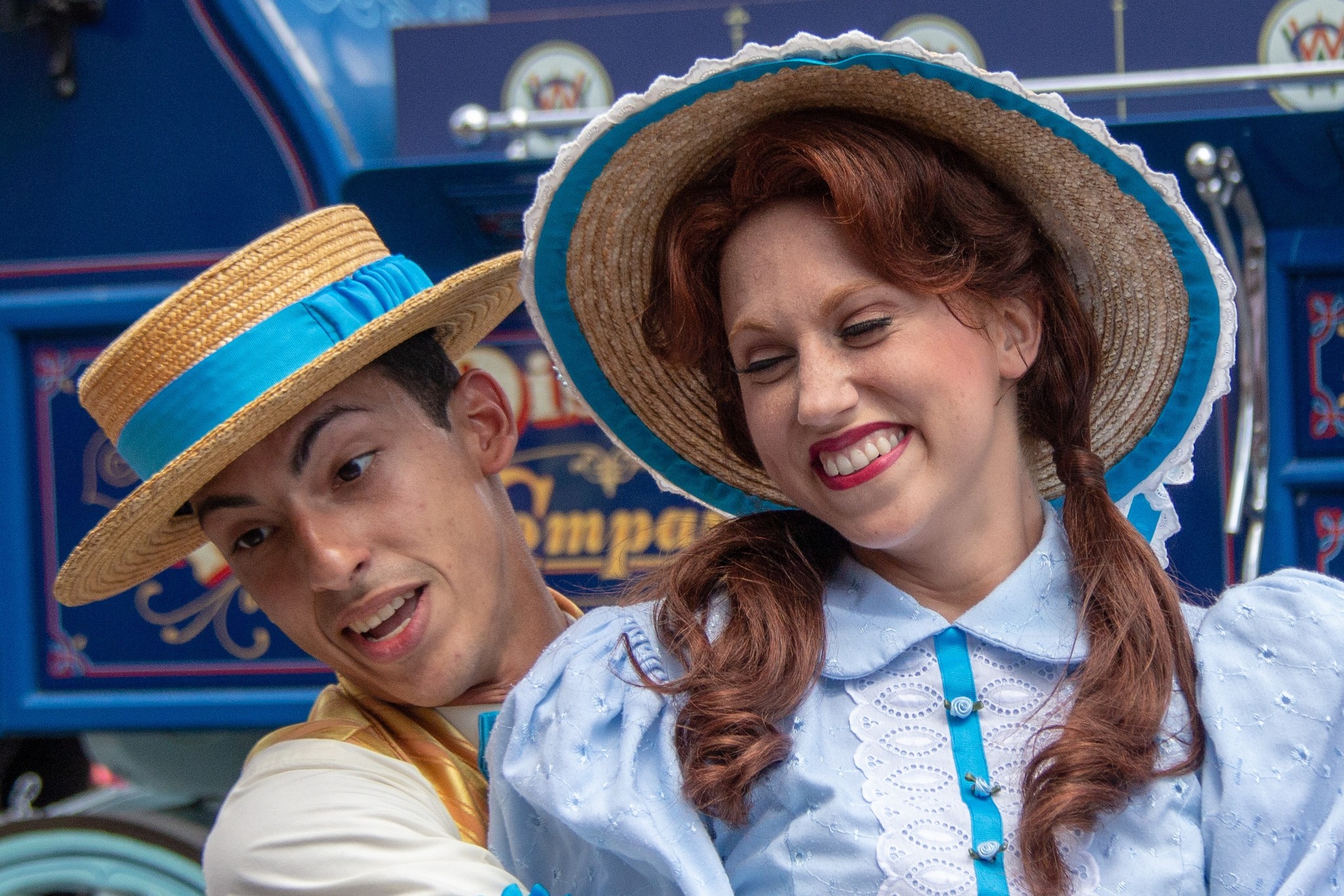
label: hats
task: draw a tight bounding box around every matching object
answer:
[50,203,535,611]
[518,29,1238,574]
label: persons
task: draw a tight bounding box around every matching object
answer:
[53,205,586,896]
[479,32,1344,896]
